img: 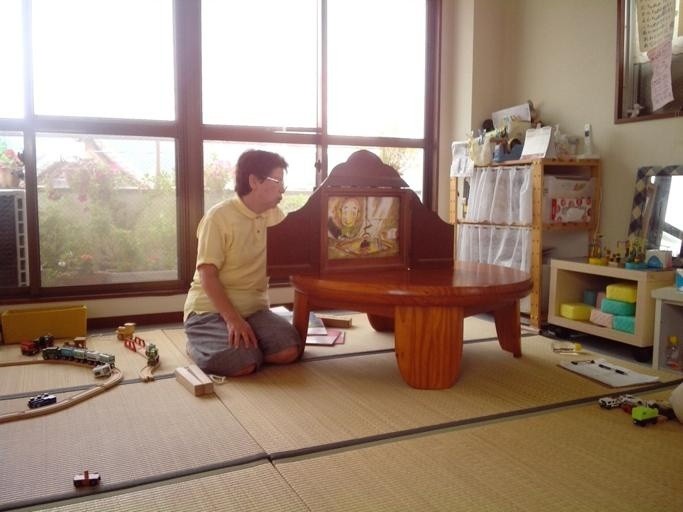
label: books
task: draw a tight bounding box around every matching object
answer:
[269,305,345,346]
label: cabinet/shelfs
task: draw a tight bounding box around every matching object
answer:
[449,159,600,329]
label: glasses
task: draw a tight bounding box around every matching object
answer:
[263,176,287,193]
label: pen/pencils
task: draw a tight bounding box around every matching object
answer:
[599,364,627,376]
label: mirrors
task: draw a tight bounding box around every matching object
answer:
[628,165,683,268]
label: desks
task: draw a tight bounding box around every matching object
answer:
[266,150,533,390]
[548,256,677,365]
[651,286,683,369]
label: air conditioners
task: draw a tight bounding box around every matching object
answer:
[0,188,30,288]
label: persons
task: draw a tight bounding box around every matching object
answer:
[180,147,304,379]
